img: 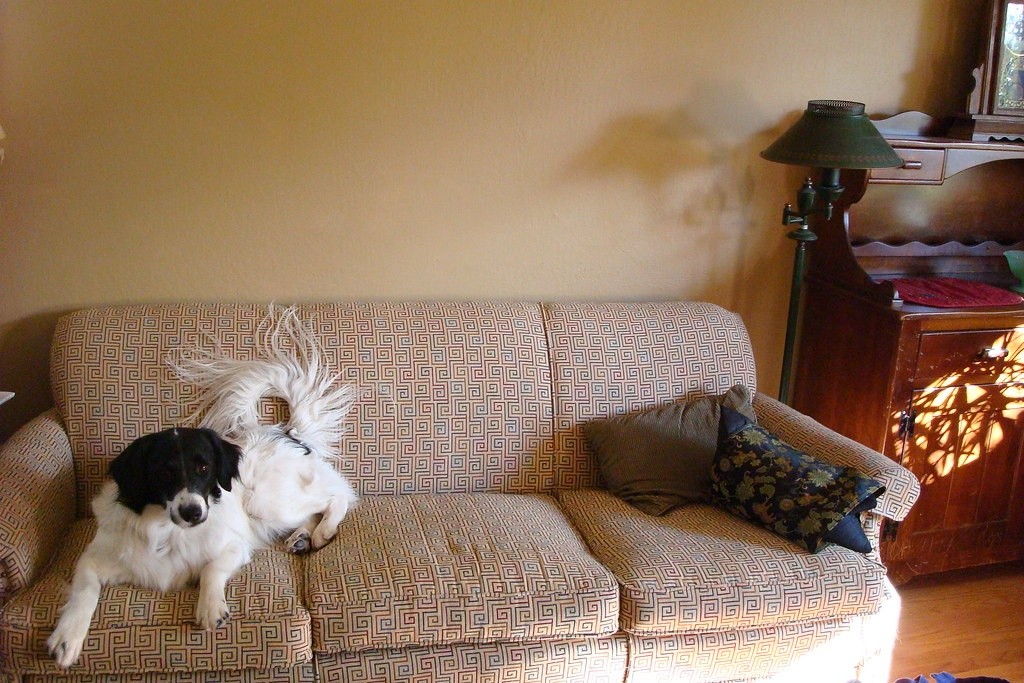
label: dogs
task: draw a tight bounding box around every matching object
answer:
[45,299,363,668]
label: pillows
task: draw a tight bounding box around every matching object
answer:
[702,404,886,555]
[584,384,757,518]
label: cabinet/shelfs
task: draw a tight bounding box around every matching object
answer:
[951,0,1024,142]
[791,110,1024,588]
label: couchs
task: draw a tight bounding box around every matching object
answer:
[0,301,920,683]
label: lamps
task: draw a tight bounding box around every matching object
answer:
[758,100,905,405]
